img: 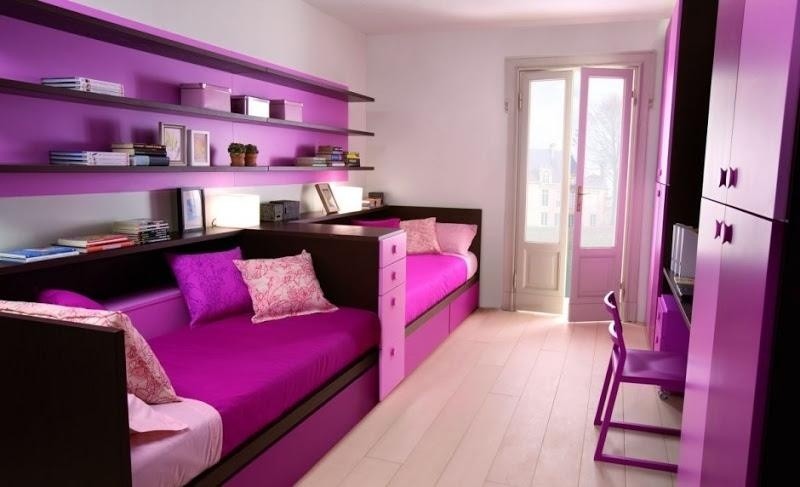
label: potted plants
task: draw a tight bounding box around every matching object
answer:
[228,141,259,167]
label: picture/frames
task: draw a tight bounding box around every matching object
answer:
[175,185,207,234]
[314,183,339,215]
[157,118,211,167]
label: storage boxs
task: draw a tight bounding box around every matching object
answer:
[178,81,304,122]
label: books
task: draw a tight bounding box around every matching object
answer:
[0,72,174,265]
[673,275,694,298]
[294,142,360,170]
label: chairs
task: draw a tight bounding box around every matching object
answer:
[595,291,691,474]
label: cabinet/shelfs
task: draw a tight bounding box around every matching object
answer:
[1,0,376,197]
